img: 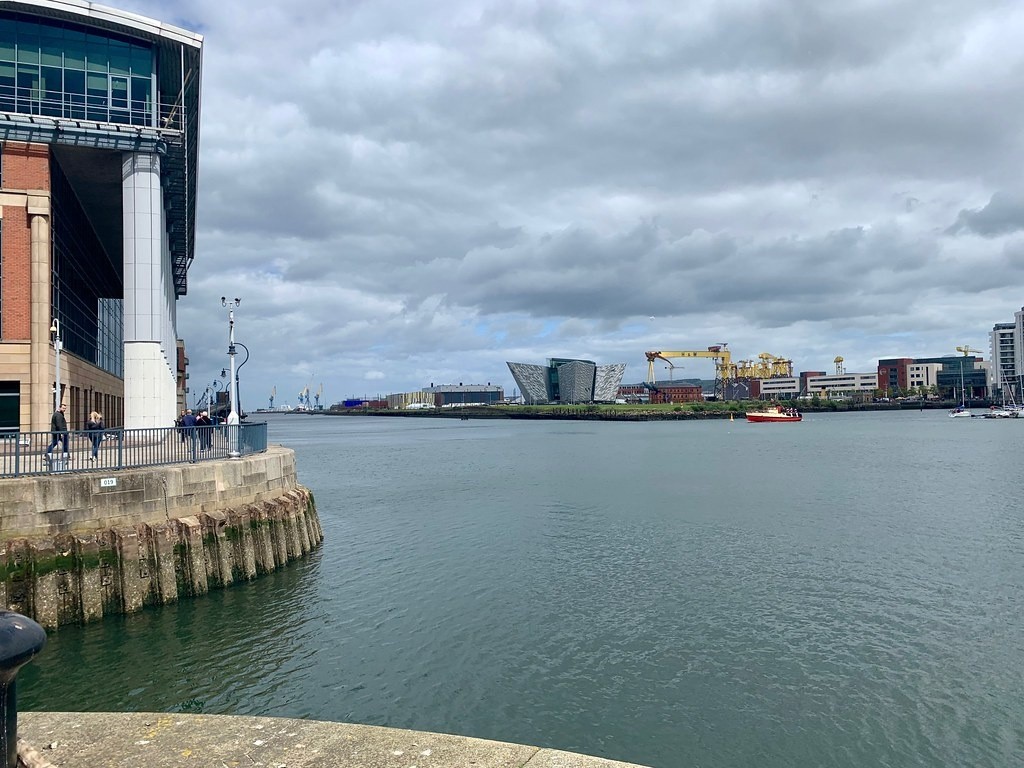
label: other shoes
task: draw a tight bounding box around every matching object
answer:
[43,457,46,461]
[90,456,95,462]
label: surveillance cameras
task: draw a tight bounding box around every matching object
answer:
[50,327,56,333]
[235,298,241,301]
[222,297,225,301]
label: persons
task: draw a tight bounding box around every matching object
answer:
[87,412,105,461]
[177,410,214,452]
[44,404,69,460]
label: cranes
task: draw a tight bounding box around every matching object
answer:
[956,344,984,356]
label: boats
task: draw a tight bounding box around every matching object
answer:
[984,367,1024,418]
[746,407,803,422]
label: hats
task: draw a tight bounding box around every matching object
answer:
[203,412,207,416]
[187,410,192,412]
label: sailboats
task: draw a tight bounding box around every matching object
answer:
[948,361,971,417]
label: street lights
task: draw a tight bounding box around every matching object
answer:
[195,382,214,419]
[49,318,61,412]
[213,379,223,430]
[220,367,231,438]
[227,341,249,450]
